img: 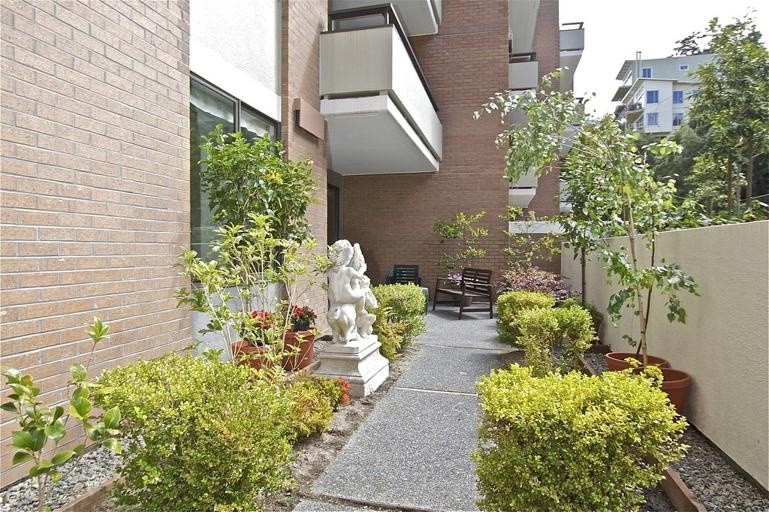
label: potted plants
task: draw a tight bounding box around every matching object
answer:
[474,65,691,435]
[431,208,489,306]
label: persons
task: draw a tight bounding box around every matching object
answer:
[324,237,369,317]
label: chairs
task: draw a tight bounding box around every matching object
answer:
[385,265,422,288]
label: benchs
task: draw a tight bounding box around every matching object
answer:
[431,268,494,319]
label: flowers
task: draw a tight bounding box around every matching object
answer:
[239,304,317,346]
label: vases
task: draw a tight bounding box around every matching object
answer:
[231,328,315,373]
